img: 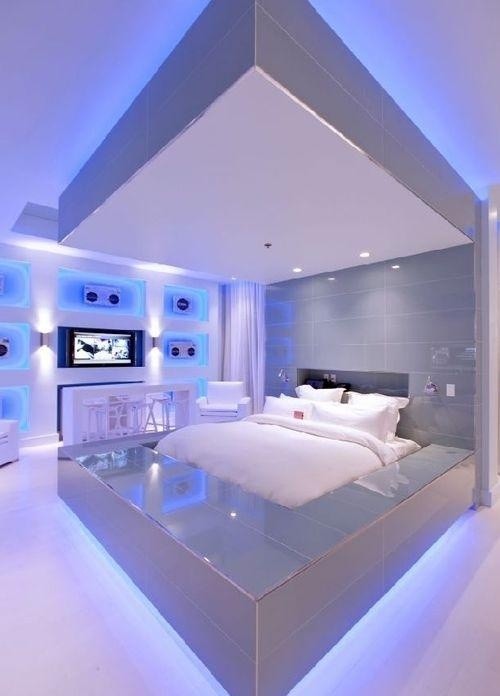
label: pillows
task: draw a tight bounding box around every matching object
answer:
[262,383,411,441]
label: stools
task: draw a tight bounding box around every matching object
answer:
[82,393,169,441]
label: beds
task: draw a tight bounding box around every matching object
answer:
[156,366,421,508]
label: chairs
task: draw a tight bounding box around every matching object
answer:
[0,418,20,467]
[194,382,252,424]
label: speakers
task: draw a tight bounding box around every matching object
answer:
[83,282,122,308]
[167,339,199,359]
[0,338,11,358]
[172,294,194,314]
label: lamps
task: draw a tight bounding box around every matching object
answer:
[424,374,439,393]
[276,368,292,383]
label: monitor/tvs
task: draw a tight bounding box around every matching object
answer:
[64,327,136,366]
